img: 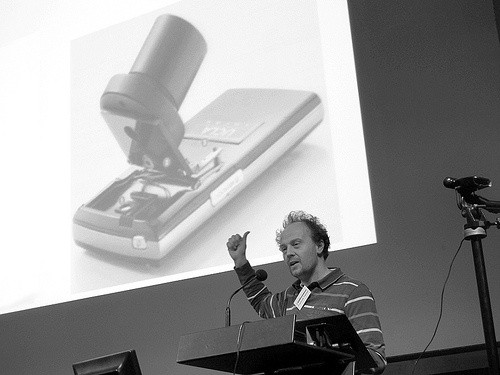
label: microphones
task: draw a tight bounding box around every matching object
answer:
[224,269,267,327]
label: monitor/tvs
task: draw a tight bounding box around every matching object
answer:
[72,348,142,375]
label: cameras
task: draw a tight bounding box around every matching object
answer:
[72,16,324,261]
[444,173,492,204]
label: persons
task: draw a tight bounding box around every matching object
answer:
[227,211,387,375]
